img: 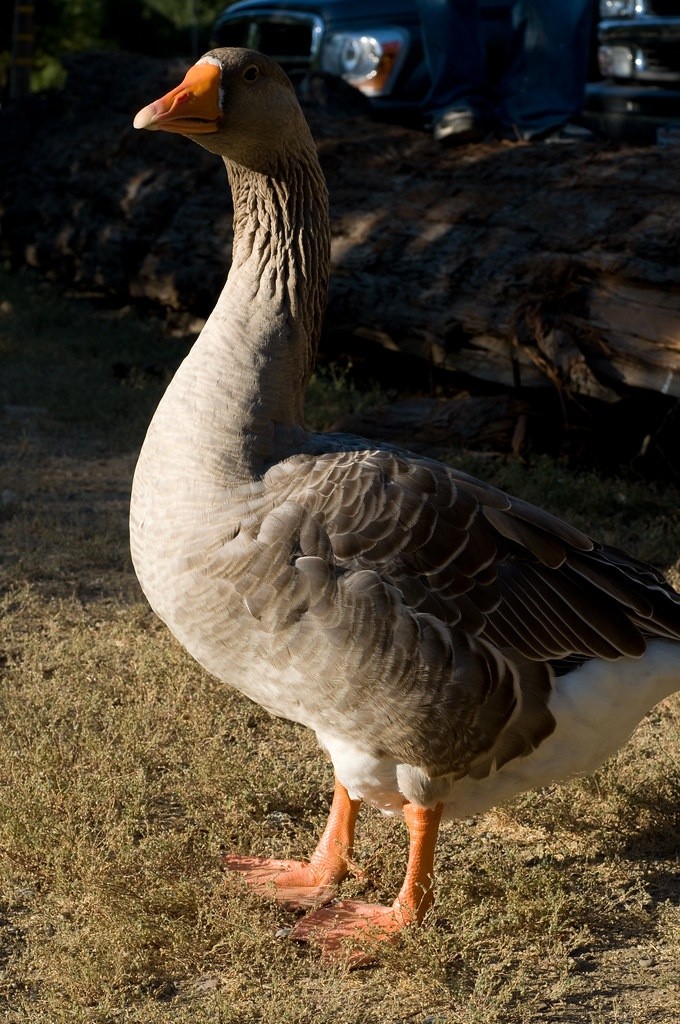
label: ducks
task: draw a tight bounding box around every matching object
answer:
[125,44,680,968]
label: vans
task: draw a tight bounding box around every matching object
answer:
[212,0,434,110]
